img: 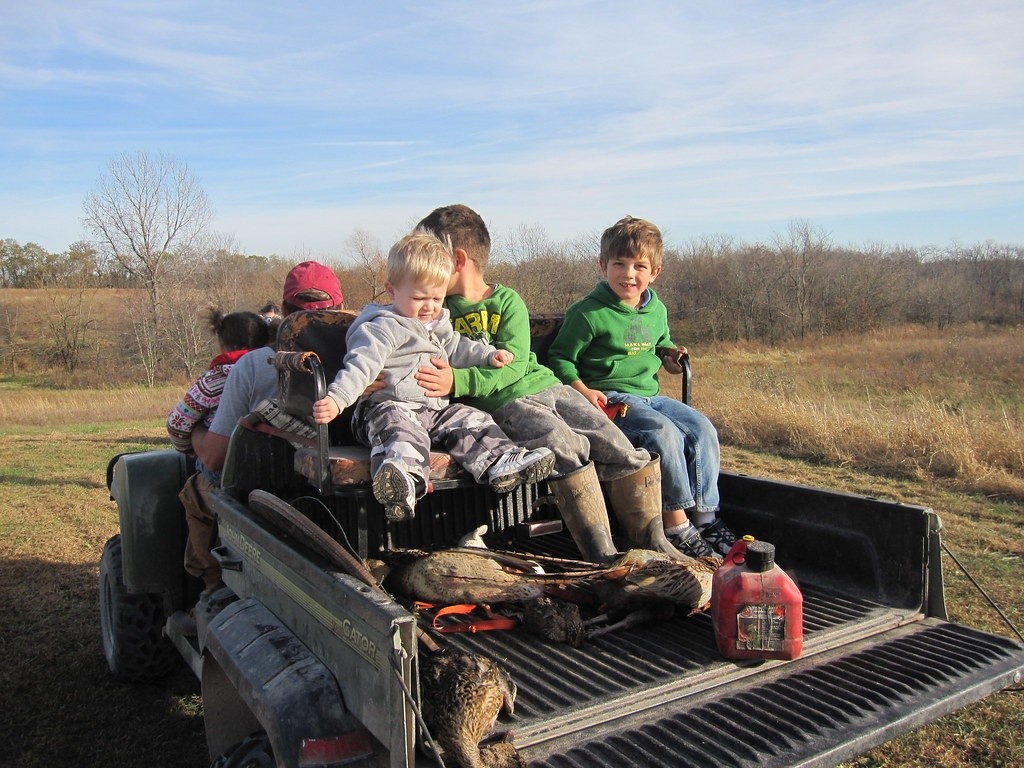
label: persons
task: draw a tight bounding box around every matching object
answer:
[546,215,740,570]
[312,224,554,523]
[359,204,709,585]
[165,263,342,649]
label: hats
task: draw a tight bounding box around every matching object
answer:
[283,261,344,310]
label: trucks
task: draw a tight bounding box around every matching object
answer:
[99,310,1023,767]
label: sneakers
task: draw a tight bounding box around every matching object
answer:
[665,522,722,561]
[697,518,736,557]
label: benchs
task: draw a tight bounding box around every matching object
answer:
[220,309,693,560]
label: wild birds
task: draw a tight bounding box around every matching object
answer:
[418,647,528,768]
[357,524,724,647]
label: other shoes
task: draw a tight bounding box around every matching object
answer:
[373,463,419,522]
[167,603,198,636]
[488,447,555,493]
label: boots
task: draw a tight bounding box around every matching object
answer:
[548,458,628,563]
[599,451,694,563]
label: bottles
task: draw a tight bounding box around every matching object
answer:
[711,536,803,660]
